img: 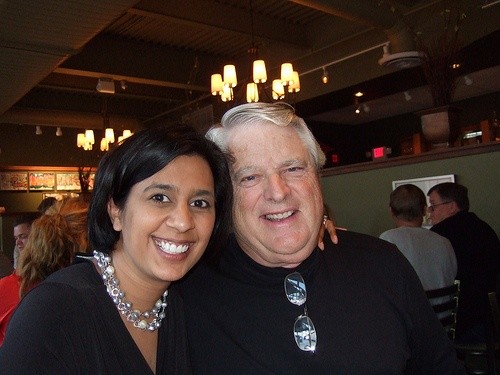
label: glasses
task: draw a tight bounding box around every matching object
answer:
[284,272,317,356]
[428,200,451,211]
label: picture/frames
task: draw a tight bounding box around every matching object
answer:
[0,171,29,191]
[28,171,56,191]
[84,171,96,192]
[55,172,83,191]
[392,174,456,230]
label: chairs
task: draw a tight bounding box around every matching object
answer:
[423,278,461,344]
[455,289,500,375]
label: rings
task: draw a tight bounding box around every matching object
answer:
[323,215,328,224]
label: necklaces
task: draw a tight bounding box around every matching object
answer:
[93,249,169,331]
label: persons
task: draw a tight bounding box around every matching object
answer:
[427,182,500,318]
[0,122,348,375]
[13,213,36,252]
[379,184,457,319]
[0,196,92,347]
[39,197,57,215]
[172,102,461,375]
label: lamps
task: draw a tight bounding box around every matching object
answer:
[76,94,134,156]
[321,67,330,85]
[55,127,63,137]
[403,91,412,102]
[35,126,42,136]
[464,75,472,86]
[210,0,301,109]
